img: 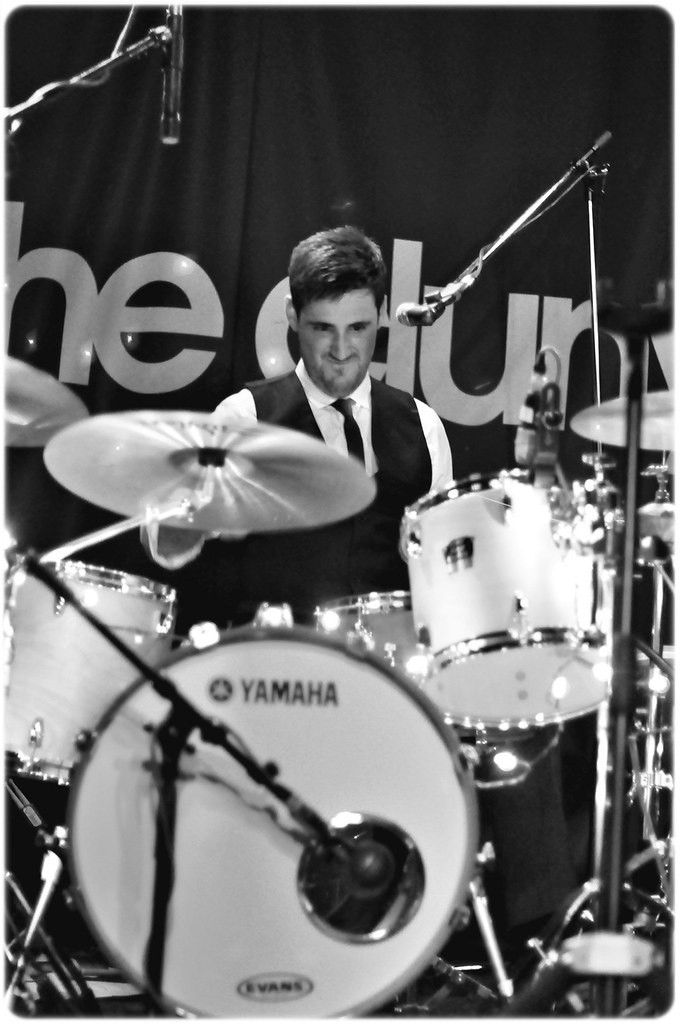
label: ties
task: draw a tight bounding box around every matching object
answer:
[330,397,364,465]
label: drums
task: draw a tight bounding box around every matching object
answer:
[398,469,613,728]
[313,587,419,677]
[4,554,176,789]
[65,626,481,1018]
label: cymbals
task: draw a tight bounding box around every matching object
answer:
[4,354,91,449]
[566,389,674,451]
[42,409,378,534]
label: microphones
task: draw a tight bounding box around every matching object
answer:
[157,5,192,173]
[396,302,434,327]
[514,361,547,465]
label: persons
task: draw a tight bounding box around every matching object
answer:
[142,228,452,631]
